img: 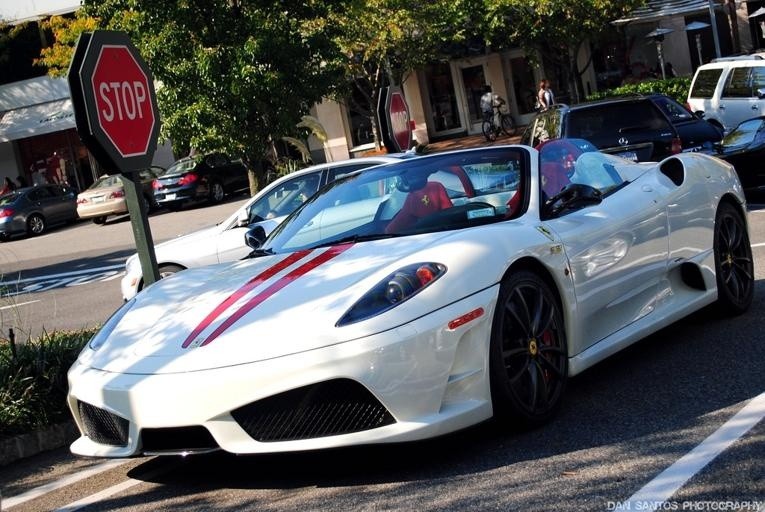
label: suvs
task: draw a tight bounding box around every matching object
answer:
[687,54,765,134]
[519,95,720,172]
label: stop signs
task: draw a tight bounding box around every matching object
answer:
[77,29,158,173]
[389,89,415,152]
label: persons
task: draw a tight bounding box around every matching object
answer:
[479,85,505,140]
[0,176,16,196]
[665,62,679,79]
[30,163,48,187]
[537,78,556,112]
[16,176,29,189]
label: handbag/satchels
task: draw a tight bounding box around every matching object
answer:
[535,95,543,112]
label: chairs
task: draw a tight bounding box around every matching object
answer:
[500,162,575,219]
[385,180,454,236]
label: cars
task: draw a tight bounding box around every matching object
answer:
[151,150,278,208]
[0,184,83,239]
[679,114,765,195]
[119,152,520,302]
[75,165,165,225]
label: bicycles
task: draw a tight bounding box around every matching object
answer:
[481,105,518,144]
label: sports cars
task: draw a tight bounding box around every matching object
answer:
[65,141,755,460]
